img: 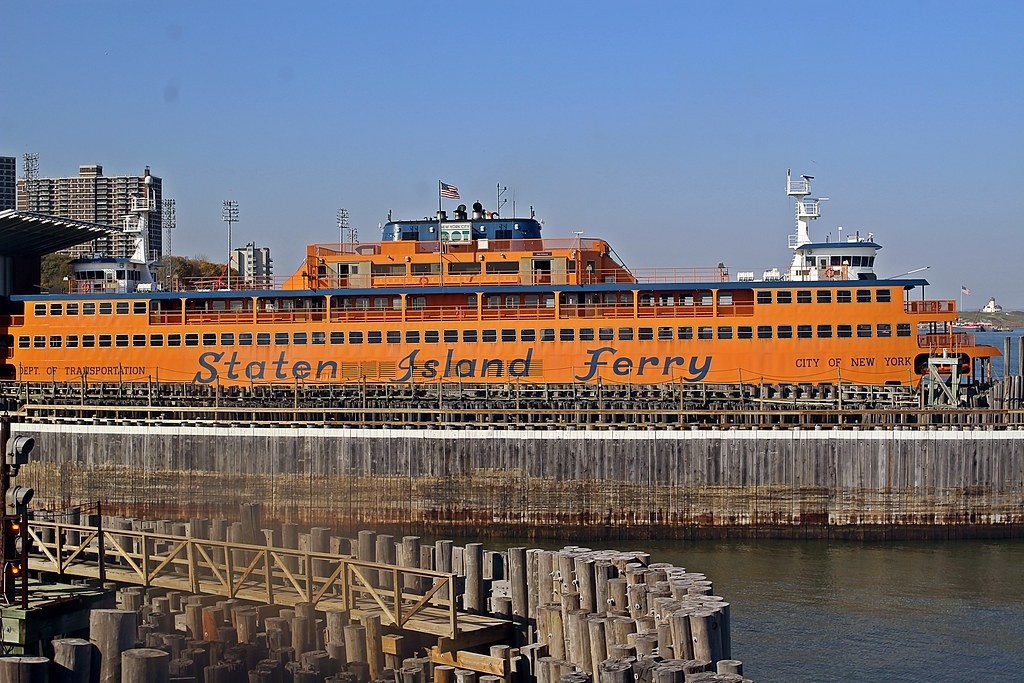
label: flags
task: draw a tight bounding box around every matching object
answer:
[440,181,461,199]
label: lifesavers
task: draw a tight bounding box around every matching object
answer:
[82,283,90,292]
[932,302,941,310]
[456,310,465,318]
[419,277,428,285]
[824,268,834,277]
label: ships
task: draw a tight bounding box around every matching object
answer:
[0,170,1023,387]
